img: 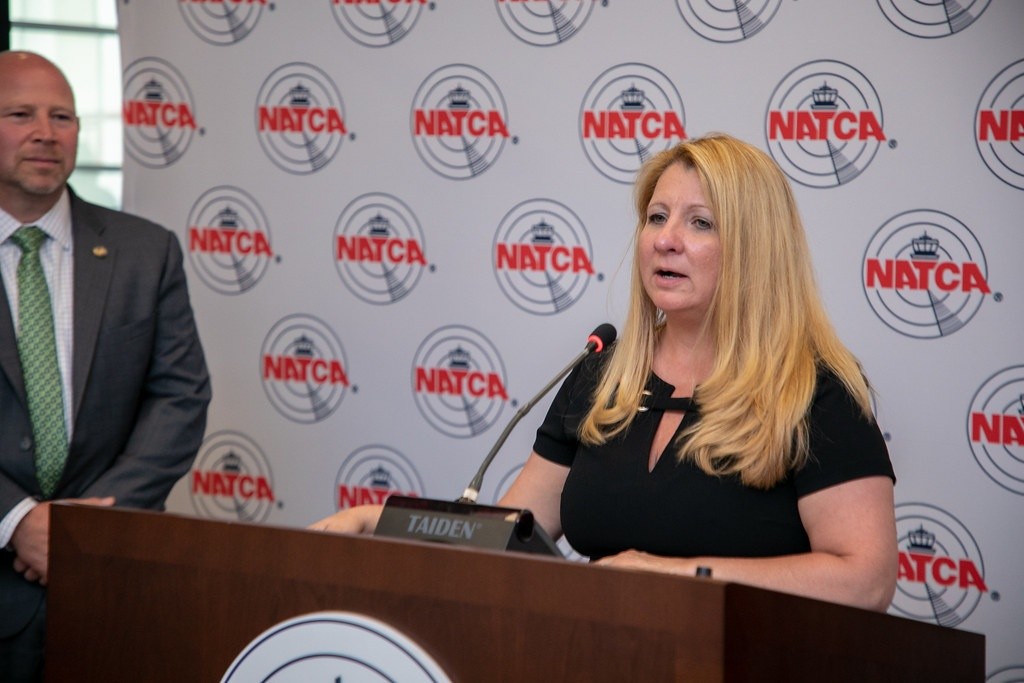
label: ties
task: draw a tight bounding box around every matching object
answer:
[11,226,68,500]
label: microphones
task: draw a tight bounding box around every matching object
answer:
[372,323,617,559]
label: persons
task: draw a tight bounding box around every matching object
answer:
[0,50,213,683]
[310,135,899,611]
